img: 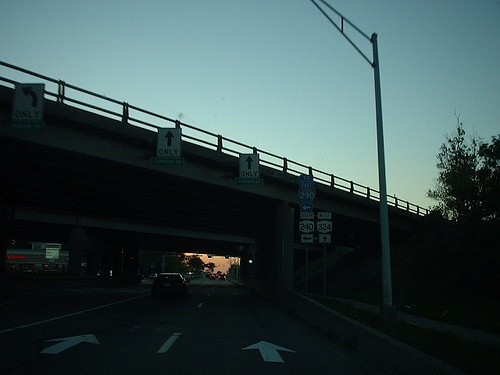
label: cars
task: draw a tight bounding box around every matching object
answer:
[151,272,192,296]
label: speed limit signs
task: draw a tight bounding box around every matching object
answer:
[300,180,316,203]
[298,220,334,233]
